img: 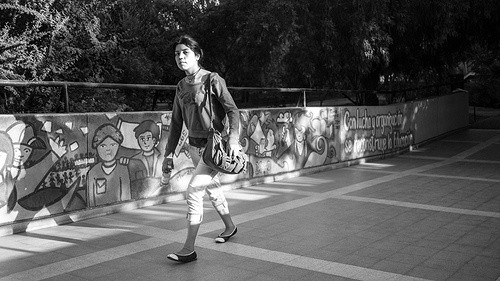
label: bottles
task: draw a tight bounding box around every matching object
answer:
[160,161,172,188]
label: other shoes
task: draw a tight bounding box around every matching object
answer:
[167,251,198,262]
[216,225,237,242]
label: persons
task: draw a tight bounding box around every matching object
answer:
[160,35,242,262]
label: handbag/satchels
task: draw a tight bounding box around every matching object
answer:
[202,129,246,174]
